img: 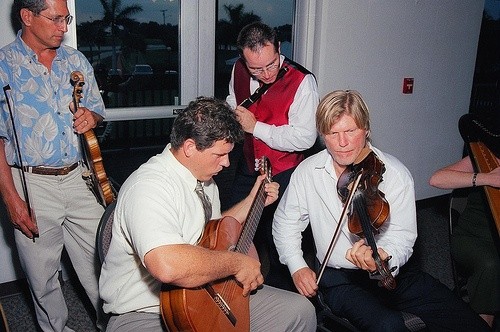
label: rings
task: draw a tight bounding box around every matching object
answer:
[85,120,88,125]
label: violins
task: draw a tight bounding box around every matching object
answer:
[336,153,398,291]
[69,70,114,207]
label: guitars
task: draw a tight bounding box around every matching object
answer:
[160,155,275,332]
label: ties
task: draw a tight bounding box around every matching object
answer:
[259,81,263,88]
[195,180,213,244]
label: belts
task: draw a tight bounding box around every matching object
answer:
[9,160,80,176]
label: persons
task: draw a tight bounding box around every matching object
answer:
[226,22,320,292]
[98,96,318,332]
[429,152,500,332]
[271,89,494,332]
[0,0,108,332]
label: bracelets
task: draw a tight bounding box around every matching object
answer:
[473,173,478,187]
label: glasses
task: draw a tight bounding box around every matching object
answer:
[251,54,281,76]
[31,10,73,27]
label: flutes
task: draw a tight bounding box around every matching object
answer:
[240,65,289,109]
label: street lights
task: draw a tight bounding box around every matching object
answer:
[160,10,168,24]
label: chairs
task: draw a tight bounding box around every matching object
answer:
[447,184,474,299]
[96,199,118,266]
[309,234,428,332]
